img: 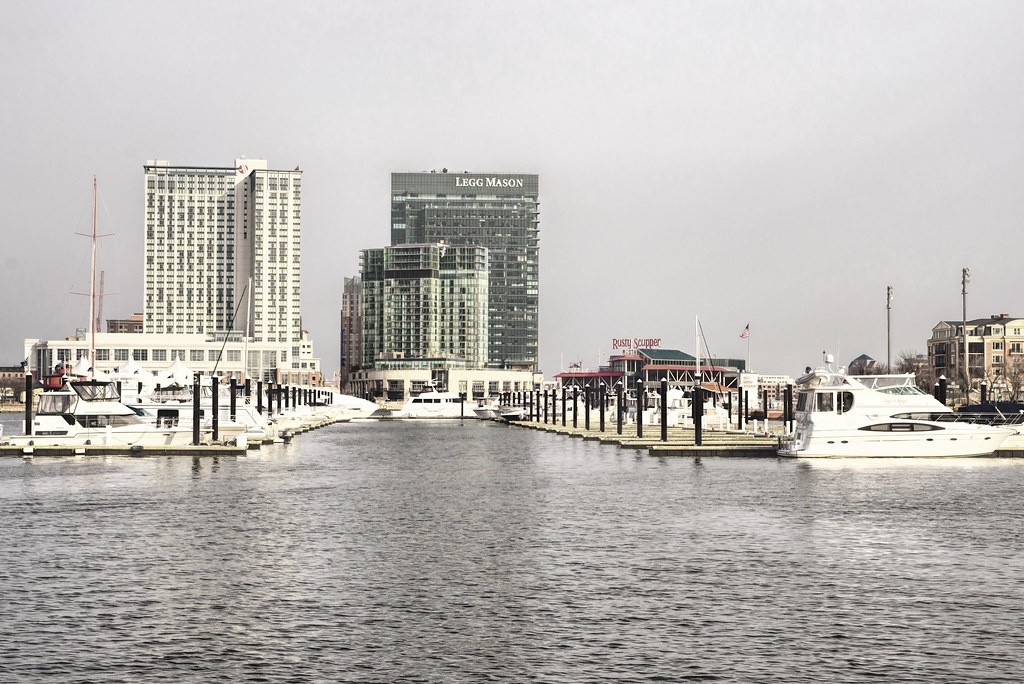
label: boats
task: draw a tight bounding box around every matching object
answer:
[472,395,744,432]
[774,335,1012,460]
[391,384,483,419]
[22,170,394,448]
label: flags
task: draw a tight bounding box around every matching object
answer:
[739,325,749,338]
